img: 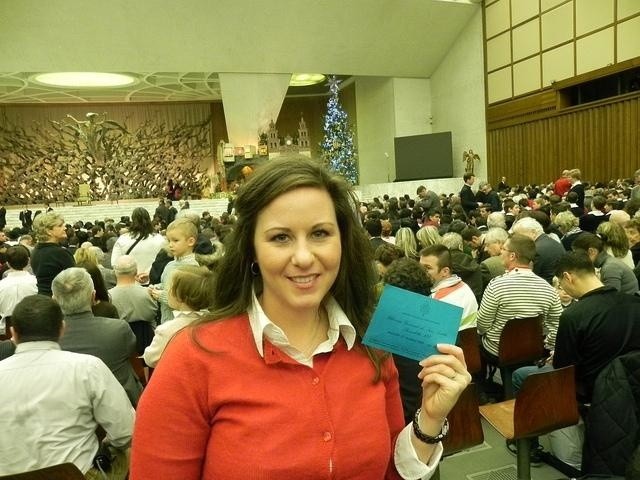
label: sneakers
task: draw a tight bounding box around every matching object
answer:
[506,437,544,469]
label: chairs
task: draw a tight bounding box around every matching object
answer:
[441,327,484,457]
[77,183,92,206]
[478,365,580,480]
[488,315,548,400]
[0,462,86,480]
[56,195,65,207]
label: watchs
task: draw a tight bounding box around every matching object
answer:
[414,409,450,443]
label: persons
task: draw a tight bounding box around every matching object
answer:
[151,214,214,283]
[153,214,167,228]
[366,221,386,249]
[4,231,20,245]
[513,217,565,284]
[0,295,136,477]
[144,267,218,366]
[202,212,210,219]
[396,226,420,262]
[481,227,533,282]
[178,199,187,211]
[419,246,478,330]
[67,217,130,252]
[572,232,639,294]
[442,232,482,304]
[156,197,169,219]
[44,203,53,213]
[381,220,396,247]
[76,260,118,317]
[107,256,157,381]
[132,157,471,480]
[52,267,143,405]
[359,169,639,226]
[1,244,39,334]
[555,212,582,254]
[149,219,199,324]
[152,221,162,233]
[478,234,561,364]
[111,208,164,273]
[597,221,634,269]
[0,203,6,230]
[0,232,7,244]
[165,199,178,221]
[91,247,117,289]
[416,225,442,247]
[552,274,577,308]
[552,249,640,402]
[31,212,75,294]
[20,204,32,226]
[624,217,640,264]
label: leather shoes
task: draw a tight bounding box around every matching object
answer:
[94,458,112,475]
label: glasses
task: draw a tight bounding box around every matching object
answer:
[498,246,518,259]
